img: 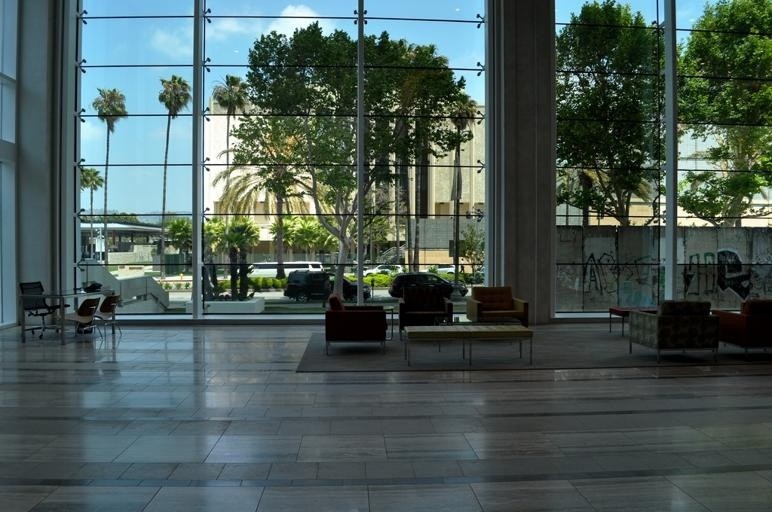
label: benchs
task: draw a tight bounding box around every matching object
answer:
[403,320,536,369]
[608,305,658,338]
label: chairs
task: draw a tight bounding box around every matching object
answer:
[397,284,455,343]
[623,298,723,369]
[708,296,771,363]
[16,279,123,344]
[463,284,534,332]
[321,291,389,358]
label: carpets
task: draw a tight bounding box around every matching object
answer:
[291,328,772,374]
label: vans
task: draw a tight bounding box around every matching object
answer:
[237,261,325,278]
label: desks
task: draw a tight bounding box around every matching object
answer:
[434,317,523,352]
[377,303,395,340]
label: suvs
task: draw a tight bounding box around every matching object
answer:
[284,270,371,306]
[363,265,403,278]
[420,264,456,275]
[387,271,469,300]
